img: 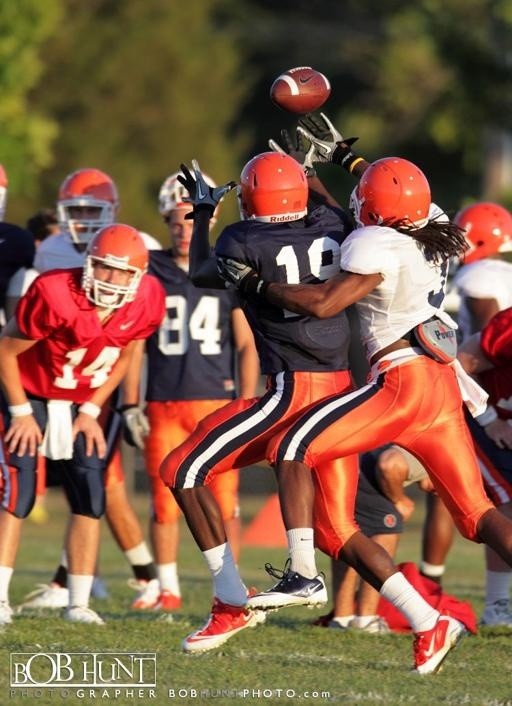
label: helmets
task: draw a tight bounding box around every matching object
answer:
[82,224,149,310]
[57,169,118,253]
[240,152,308,224]
[158,171,224,223]
[452,203,512,265]
[350,158,430,234]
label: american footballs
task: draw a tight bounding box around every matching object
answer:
[270,67,331,114]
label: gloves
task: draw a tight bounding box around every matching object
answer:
[268,129,317,178]
[296,111,365,175]
[177,159,238,220]
[216,257,275,297]
[117,402,151,452]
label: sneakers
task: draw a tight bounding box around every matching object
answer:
[247,557,328,612]
[481,604,512,630]
[348,616,391,634]
[1,601,14,626]
[62,607,105,627]
[181,586,266,654]
[326,618,351,629]
[20,583,70,608]
[409,613,466,674]
[127,578,160,609]
[147,590,182,610]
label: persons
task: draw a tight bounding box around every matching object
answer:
[0,106,512,679]
